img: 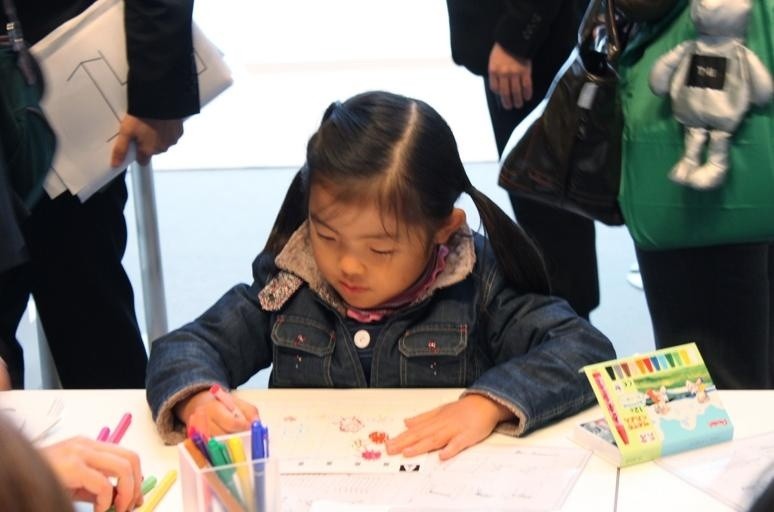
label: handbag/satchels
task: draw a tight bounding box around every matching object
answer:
[498,37,623,227]
[0,40,56,210]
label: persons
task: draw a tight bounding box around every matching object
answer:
[143,91,617,461]
[444,0,601,329]
[2,1,56,390]
[0,411,83,511]
[36,434,144,511]
[17,1,202,389]
[0,356,13,390]
[616,0,774,391]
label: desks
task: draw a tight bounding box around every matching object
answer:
[0,387,774,512]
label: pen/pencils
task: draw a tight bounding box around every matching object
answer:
[185,420,269,512]
[210,382,247,425]
[575,416,621,470]
[97,413,177,511]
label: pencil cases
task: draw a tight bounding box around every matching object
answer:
[575,342,734,470]
